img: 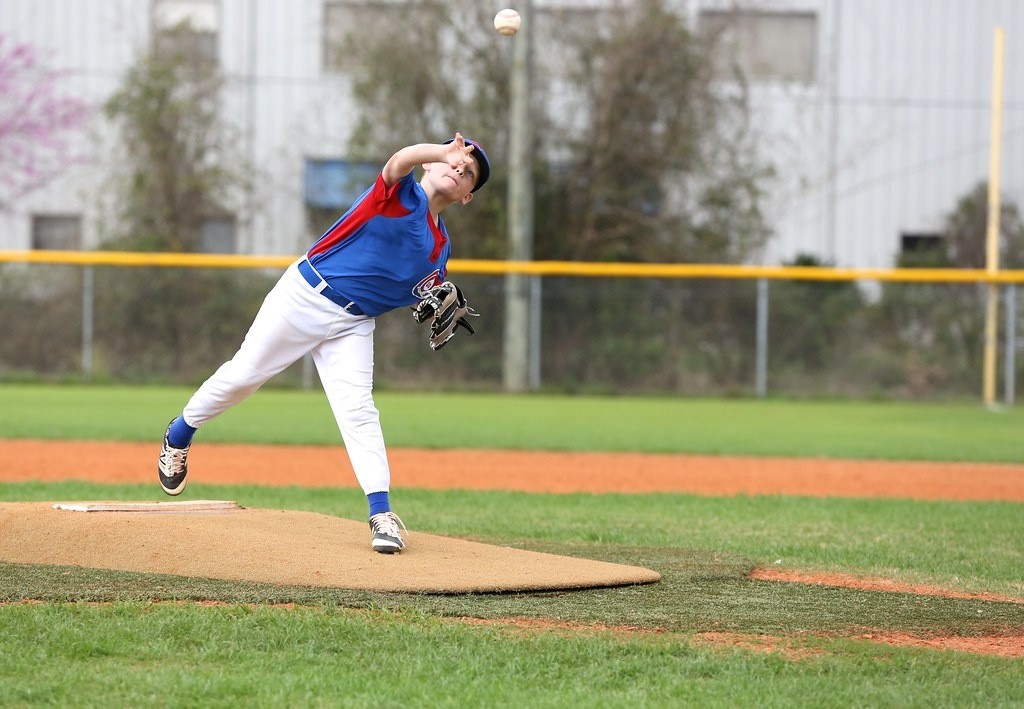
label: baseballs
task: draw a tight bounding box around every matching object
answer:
[493,9,522,36]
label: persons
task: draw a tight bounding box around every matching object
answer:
[158,132,490,553]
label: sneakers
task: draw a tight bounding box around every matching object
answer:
[158,417,194,496]
[368,511,409,553]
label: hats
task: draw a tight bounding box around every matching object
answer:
[442,137,491,194]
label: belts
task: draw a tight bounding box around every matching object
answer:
[297,260,367,316]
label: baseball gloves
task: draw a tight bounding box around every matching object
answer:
[412,279,477,352]
